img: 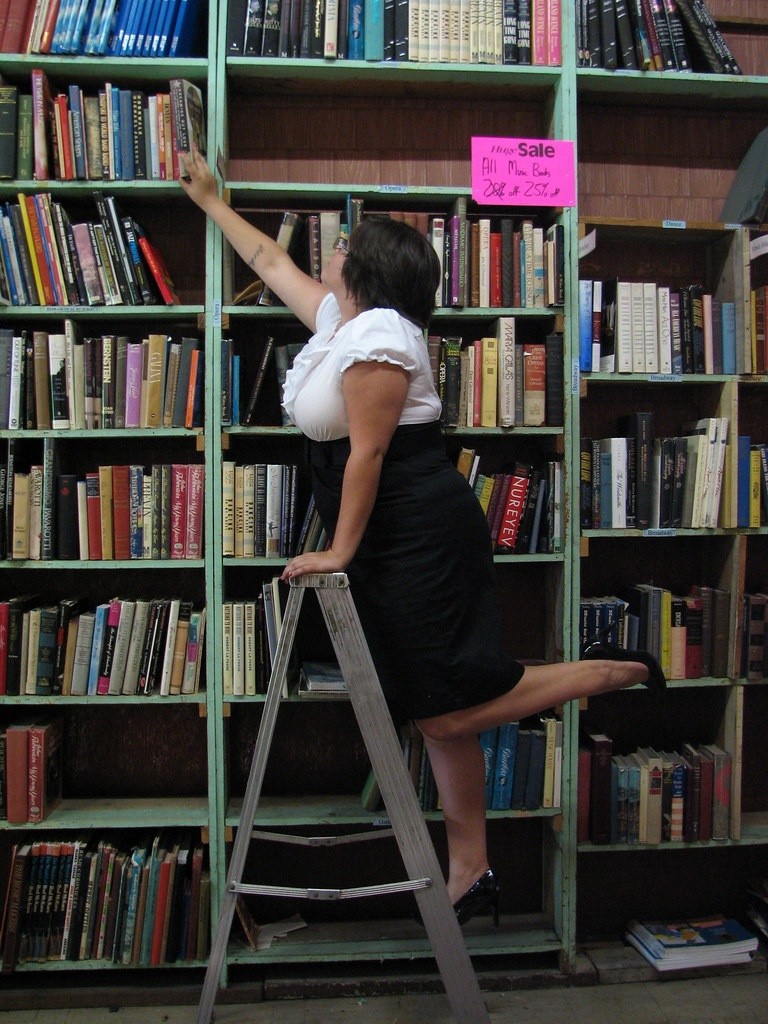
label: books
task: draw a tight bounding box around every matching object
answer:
[2,0,766,974]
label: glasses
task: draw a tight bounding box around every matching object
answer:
[333,237,349,253]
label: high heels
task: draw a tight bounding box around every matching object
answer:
[411,868,501,927]
[583,620,667,696]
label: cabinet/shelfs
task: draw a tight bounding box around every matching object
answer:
[0,0,768,995]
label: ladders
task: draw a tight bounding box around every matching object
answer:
[193,568,495,1024]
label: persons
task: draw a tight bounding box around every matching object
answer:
[177,144,666,940]
